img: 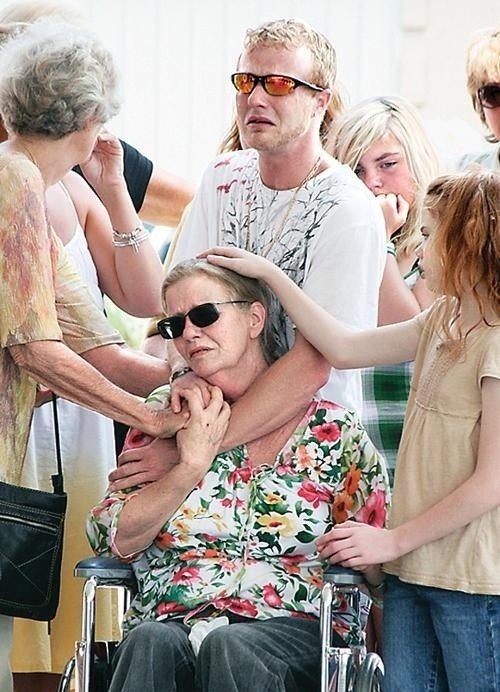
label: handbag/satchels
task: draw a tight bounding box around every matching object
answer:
[0,472,67,623]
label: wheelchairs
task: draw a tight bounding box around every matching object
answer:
[60,554,387,692]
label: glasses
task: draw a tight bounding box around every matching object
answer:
[155,299,257,341]
[230,71,323,97]
